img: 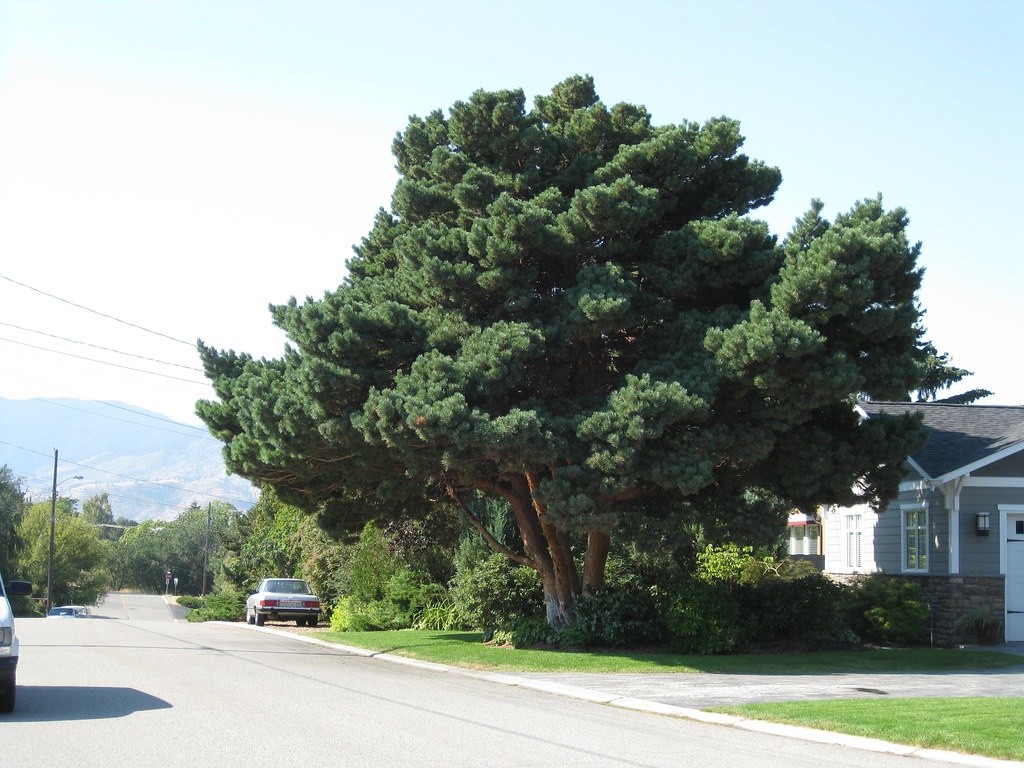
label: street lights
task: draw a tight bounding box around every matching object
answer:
[46,476,82,612]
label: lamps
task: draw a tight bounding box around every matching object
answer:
[977,512,990,535]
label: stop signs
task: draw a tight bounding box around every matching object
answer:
[165,572,171,579]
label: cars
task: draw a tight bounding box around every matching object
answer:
[0,571,34,713]
[46,605,80,619]
[71,606,89,618]
[246,576,321,627]
